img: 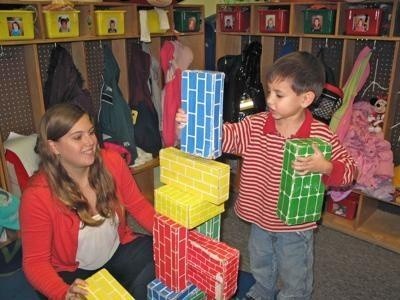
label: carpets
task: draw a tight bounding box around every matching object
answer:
[1,269,255,300]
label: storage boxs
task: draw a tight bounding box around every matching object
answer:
[327,191,360,220]
[1,10,201,40]
[220,8,383,35]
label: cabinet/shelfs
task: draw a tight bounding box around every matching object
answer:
[214,3,400,255]
[1,4,204,207]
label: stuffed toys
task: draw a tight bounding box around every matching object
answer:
[367,94,388,134]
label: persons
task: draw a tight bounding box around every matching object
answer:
[311,18,321,32]
[58,17,70,32]
[108,19,117,33]
[10,22,23,36]
[175,51,358,300]
[225,19,232,29]
[354,18,364,32]
[266,18,275,29]
[19,104,157,300]
